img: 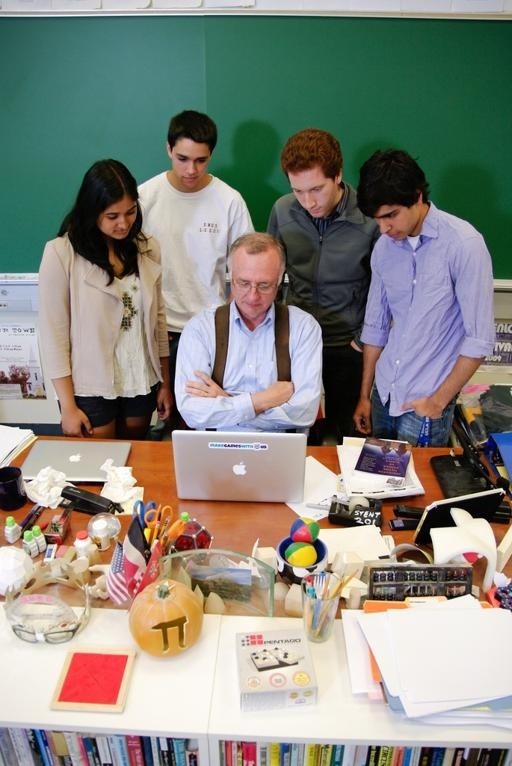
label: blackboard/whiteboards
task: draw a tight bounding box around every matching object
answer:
[1,12,512,292]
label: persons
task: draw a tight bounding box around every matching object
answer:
[266,127,382,446]
[175,232,323,437]
[353,148,495,447]
[37,159,175,438]
[137,110,256,431]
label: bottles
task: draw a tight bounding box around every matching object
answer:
[4,516,47,557]
[75,519,112,563]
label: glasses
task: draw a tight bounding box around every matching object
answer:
[238,281,275,295]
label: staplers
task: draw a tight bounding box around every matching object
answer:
[389,505,424,530]
[59,486,115,515]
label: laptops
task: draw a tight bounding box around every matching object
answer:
[171,429,308,503]
[20,439,132,484]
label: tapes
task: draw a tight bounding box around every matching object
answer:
[348,496,370,512]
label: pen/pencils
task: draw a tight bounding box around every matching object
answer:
[18,504,45,538]
[305,569,359,638]
[150,519,168,547]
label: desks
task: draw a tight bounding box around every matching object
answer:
[0,434,509,619]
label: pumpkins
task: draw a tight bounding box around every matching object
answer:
[129,578,204,659]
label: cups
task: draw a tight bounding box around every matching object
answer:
[0,466,29,511]
[301,572,342,642]
[277,537,328,587]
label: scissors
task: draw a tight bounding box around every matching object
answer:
[134,500,173,531]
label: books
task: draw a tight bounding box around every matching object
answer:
[0,729,200,766]
[220,740,345,766]
[335,437,426,500]
[354,745,510,766]
[341,594,512,732]
[0,424,38,469]
